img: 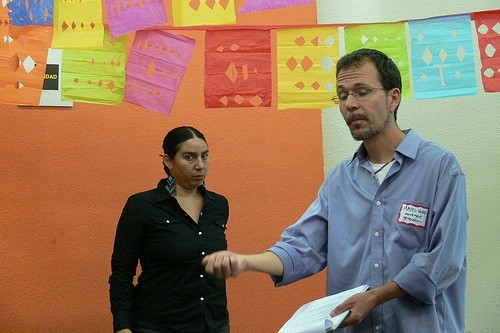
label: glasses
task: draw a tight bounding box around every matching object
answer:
[331,87,388,104]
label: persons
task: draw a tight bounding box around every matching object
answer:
[201,48,467,333]
[107,126,230,333]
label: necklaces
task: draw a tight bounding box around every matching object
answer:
[375,157,395,174]
